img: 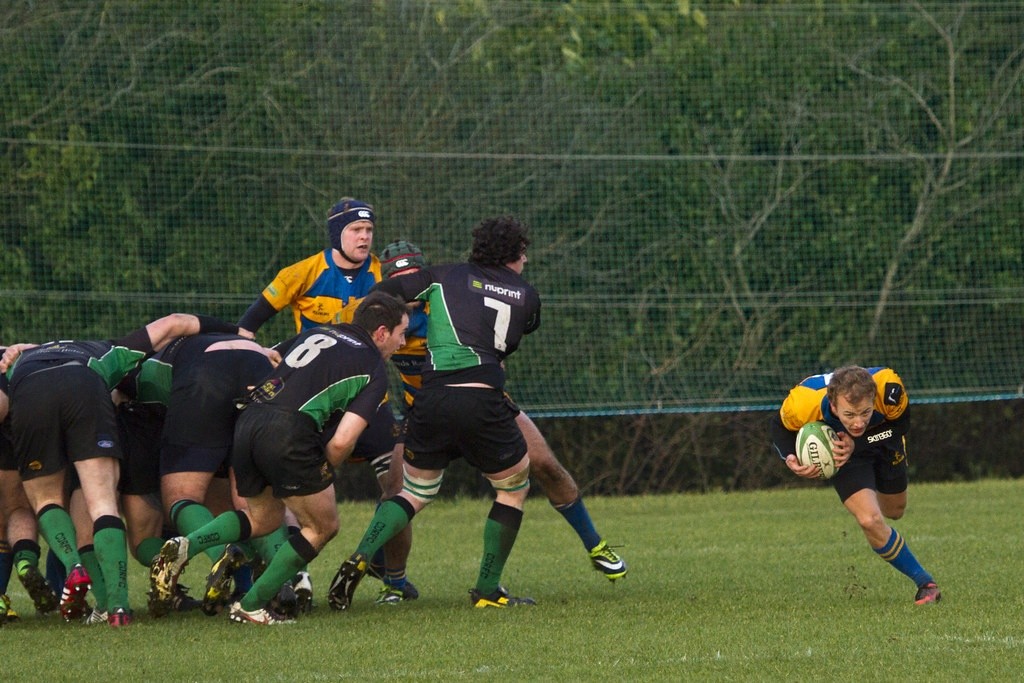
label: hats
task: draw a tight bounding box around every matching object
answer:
[326,198,376,248]
[379,240,432,279]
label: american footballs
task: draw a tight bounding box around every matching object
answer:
[796,421,841,481]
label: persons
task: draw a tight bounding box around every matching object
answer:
[238,195,418,600]
[771,365,941,605]
[328,217,541,613]
[0,290,410,629]
[373,241,628,609]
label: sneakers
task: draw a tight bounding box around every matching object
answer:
[201,544,248,616]
[383,575,418,600]
[81,605,107,626]
[366,560,386,580]
[375,585,402,607]
[107,606,132,626]
[467,585,537,609]
[914,582,940,605]
[0,594,17,623]
[16,558,57,612]
[588,538,628,581]
[148,536,188,604]
[169,583,198,610]
[290,571,314,612]
[251,568,295,618]
[230,600,296,625]
[327,560,364,612]
[60,563,92,621]
